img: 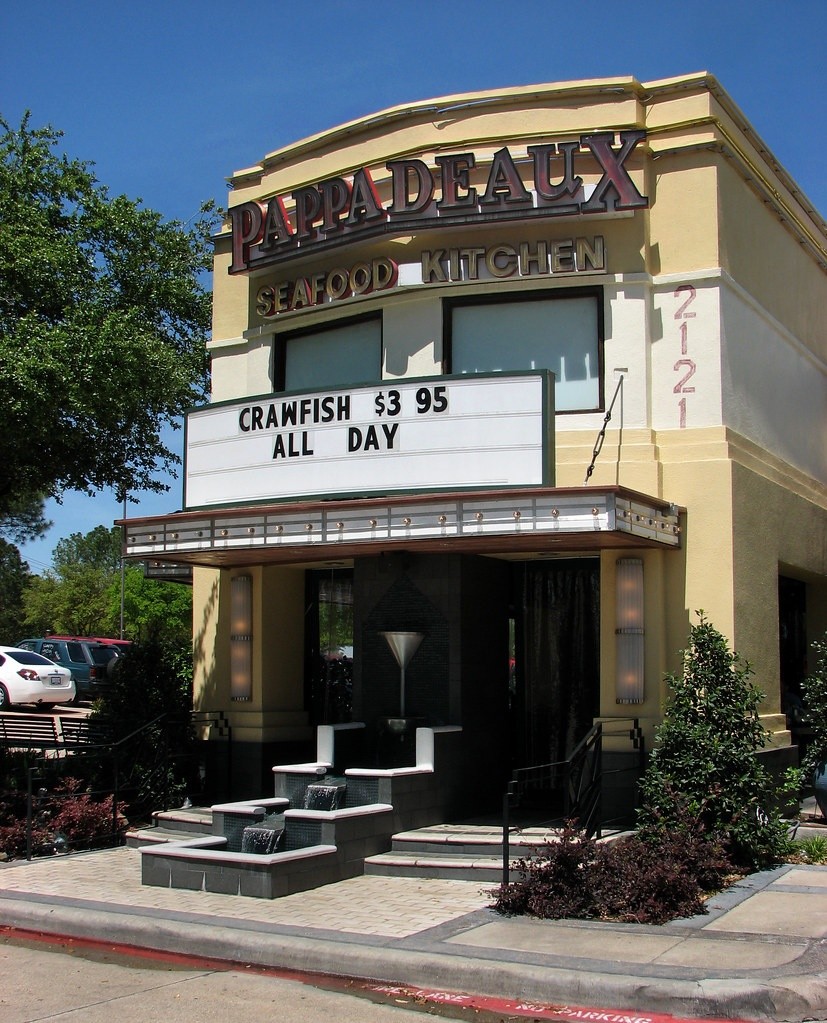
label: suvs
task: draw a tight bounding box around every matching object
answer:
[13,639,125,707]
[48,635,131,654]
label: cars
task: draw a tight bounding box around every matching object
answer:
[0,646,77,714]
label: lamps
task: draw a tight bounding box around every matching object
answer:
[614,556,643,705]
[229,575,250,701]
